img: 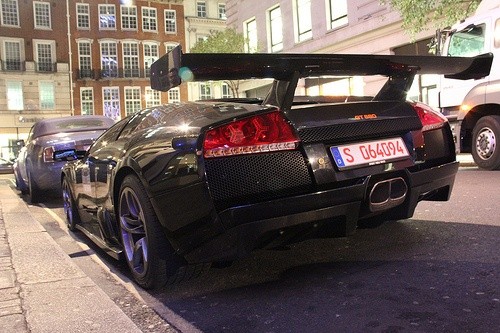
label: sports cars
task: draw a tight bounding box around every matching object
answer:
[51,43,494,291]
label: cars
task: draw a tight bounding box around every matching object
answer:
[0,157,15,174]
[456,79,500,171]
[13,114,116,204]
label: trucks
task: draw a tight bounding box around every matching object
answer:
[435,0,500,121]
